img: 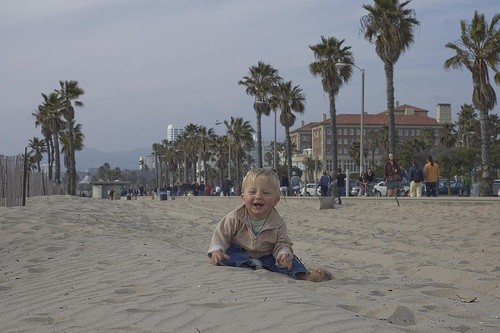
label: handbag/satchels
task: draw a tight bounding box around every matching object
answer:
[393,173,401,182]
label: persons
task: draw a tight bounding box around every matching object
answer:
[208,168,325,283]
[409,159,424,198]
[383,152,402,197]
[423,156,440,197]
[357,168,376,196]
[107,167,346,201]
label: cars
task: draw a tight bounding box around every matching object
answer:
[299,183,323,200]
[372,181,413,201]
[424,180,465,198]
[326,179,361,196]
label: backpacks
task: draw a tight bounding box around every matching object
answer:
[412,166,423,182]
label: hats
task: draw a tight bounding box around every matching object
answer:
[292,172,296,176]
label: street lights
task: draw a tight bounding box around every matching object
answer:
[216,122,232,181]
[174,150,185,192]
[336,62,365,198]
[257,100,277,172]
